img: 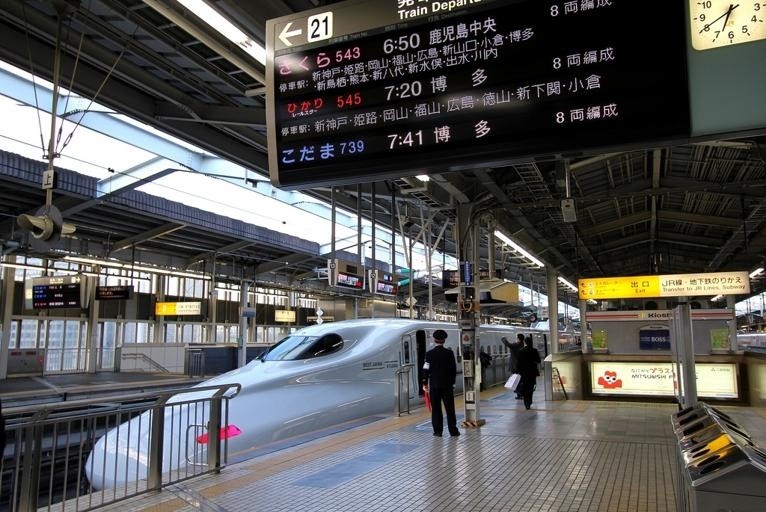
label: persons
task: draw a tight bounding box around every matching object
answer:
[500,333,525,400]
[519,336,542,410]
[420,328,461,437]
[464,344,474,360]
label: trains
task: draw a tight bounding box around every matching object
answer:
[84,316,590,489]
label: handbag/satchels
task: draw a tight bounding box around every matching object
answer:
[503,373,522,392]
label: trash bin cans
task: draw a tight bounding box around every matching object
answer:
[670,400,766,511]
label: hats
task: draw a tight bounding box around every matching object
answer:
[431,329,448,345]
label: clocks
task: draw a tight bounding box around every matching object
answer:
[689,0,766,51]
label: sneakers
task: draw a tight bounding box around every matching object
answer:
[448,431,461,438]
[431,431,442,438]
